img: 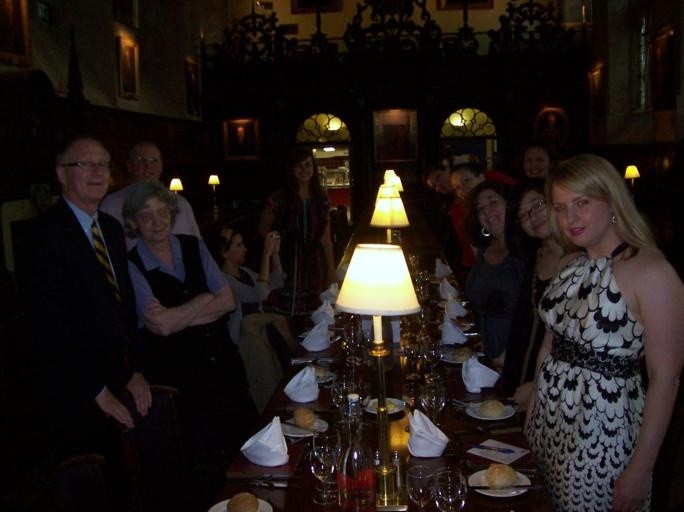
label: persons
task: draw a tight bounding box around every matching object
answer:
[523,140,554,179]
[100,140,201,254]
[452,164,487,198]
[14,127,153,429]
[122,179,262,457]
[465,182,527,375]
[213,224,297,416]
[522,153,684,512]
[504,177,586,414]
[255,150,334,330]
[421,164,520,286]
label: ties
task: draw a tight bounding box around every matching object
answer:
[89,216,123,303]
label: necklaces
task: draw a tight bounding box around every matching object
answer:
[530,246,557,315]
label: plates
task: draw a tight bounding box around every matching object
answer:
[207,275,532,512]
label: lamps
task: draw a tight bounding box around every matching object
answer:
[371,168,411,242]
[336,244,423,512]
[208,175,220,222]
[624,166,642,186]
[169,178,184,196]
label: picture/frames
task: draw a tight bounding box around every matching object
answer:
[119,37,141,100]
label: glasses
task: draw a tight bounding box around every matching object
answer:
[131,154,161,166]
[58,159,112,171]
[514,197,548,225]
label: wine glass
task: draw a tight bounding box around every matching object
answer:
[308,270,467,512]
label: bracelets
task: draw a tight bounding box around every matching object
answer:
[256,277,270,287]
